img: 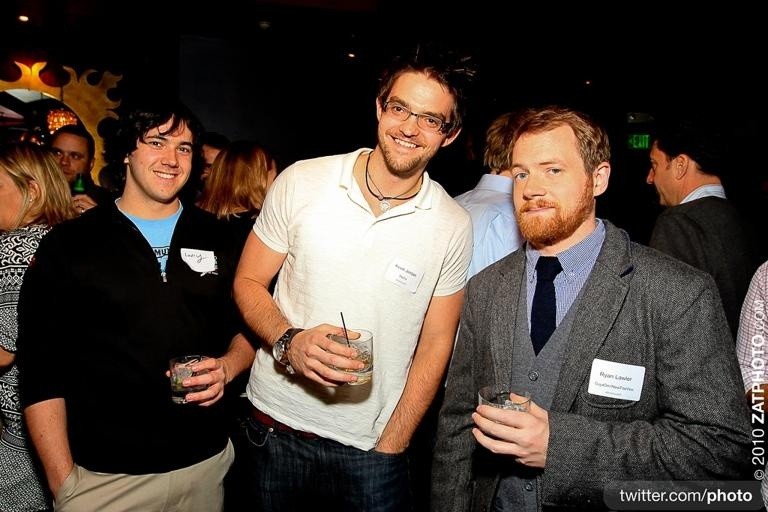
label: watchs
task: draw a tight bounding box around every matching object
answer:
[272,327,303,376]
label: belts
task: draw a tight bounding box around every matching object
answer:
[249,407,318,438]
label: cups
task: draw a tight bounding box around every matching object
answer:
[477,384,531,440]
[169,355,210,404]
[327,329,373,386]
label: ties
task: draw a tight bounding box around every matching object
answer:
[532,256,563,357]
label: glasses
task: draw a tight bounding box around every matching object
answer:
[384,99,450,134]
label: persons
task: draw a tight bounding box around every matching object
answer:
[648,123,761,350]
[1,104,277,511]
[434,111,753,512]
[446,113,530,386]
[736,259,767,510]
[220,50,472,511]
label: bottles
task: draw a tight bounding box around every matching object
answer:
[74,173,84,195]
